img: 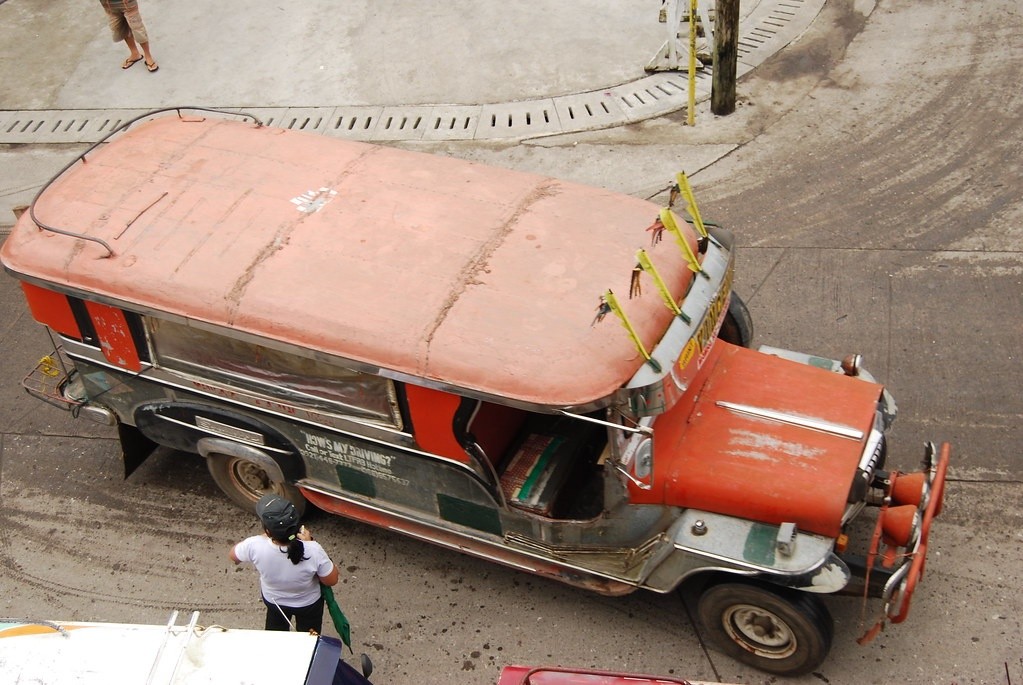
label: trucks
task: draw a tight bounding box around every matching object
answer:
[0,103,950,675]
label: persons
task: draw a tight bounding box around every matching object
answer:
[98,0,159,71]
[231,495,338,632]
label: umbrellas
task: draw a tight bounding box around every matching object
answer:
[319,579,353,655]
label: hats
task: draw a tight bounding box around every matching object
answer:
[255,493,299,535]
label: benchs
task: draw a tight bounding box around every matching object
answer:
[494,411,604,520]
